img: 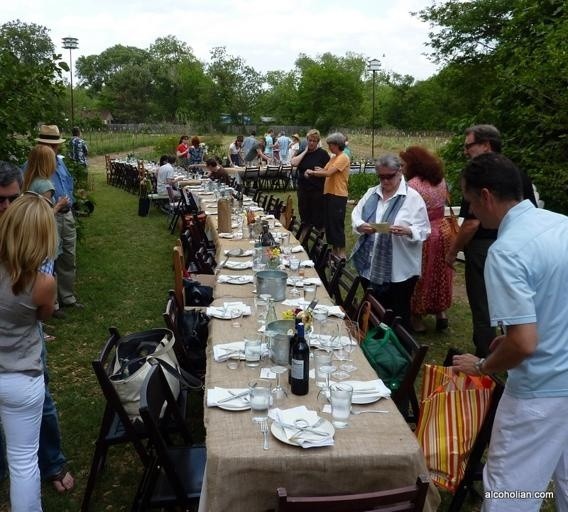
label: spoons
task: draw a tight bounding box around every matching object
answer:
[227,390,249,403]
[217,249,244,276]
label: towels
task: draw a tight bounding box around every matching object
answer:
[224,249,252,256]
[339,381,391,400]
[327,306,345,319]
[309,334,319,346]
[300,260,315,268]
[290,245,304,252]
[219,233,232,237]
[303,278,321,285]
[206,389,250,408]
[206,302,251,318]
[217,275,253,283]
[220,261,252,268]
[266,215,274,219]
[275,222,282,226]
[415,364,496,496]
[268,406,334,449]
[213,341,246,362]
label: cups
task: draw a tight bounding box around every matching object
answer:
[313,347,333,387]
[329,382,353,430]
[231,308,244,328]
[319,319,339,378]
[247,379,271,423]
[243,334,262,368]
[226,346,240,370]
[243,225,253,240]
[255,297,269,325]
[288,256,300,272]
[303,283,317,305]
[277,233,292,252]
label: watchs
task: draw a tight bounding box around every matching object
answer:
[476,358,487,376]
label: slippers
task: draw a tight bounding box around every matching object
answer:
[51,469,76,494]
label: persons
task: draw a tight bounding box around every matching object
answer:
[67,126,89,167]
[351,154,431,335]
[244,137,270,163]
[458,124,538,358]
[226,136,244,167]
[453,151,568,512]
[176,135,193,171]
[206,158,230,185]
[0,191,56,512]
[242,130,258,159]
[305,132,350,264]
[187,136,204,175]
[154,155,168,193]
[399,146,460,336]
[275,131,292,163]
[264,129,279,158]
[289,134,299,161]
[24,144,72,215]
[157,155,185,214]
[23,124,84,319]
[294,129,330,231]
[0,159,77,495]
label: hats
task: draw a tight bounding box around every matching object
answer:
[292,133,300,141]
[34,125,66,144]
[237,135,243,141]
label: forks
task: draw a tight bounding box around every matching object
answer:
[349,409,391,415]
[260,419,269,450]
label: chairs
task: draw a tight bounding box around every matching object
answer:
[392,316,430,426]
[301,223,325,255]
[277,475,430,512]
[193,213,208,242]
[276,166,293,193]
[309,236,328,268]
[172,239,214,307]
[329,262,361,311]
[289,215,307,240]
[350,162,362,175]
[262,165,280,193]
[81,326,147,512]
[364,161,375,173]
[197,242,217,275]
[242,185,260,201]
[233,180,244,192]
[165,186,185,224]
[275,200,287,221]
[317,248,346,294]
[105,155,159,196]
[173,164,203,185]
[226,174,236,188]
[167,188,195,234]
[442,348,505,512]
[146,179,175,216]
[352,288,394,336]
[180,225,202,270]
[257,191,269,210]
[266,195,280,215]
[242,166,262,189]
[131,364,208,512]
[163,288,207,375]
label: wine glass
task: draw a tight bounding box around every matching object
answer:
[340,320,359,379]
[316,365,337,420]
[312,307,328,344]
[255,319,272,359]
[289,270,301,294]
[270,365,289,410]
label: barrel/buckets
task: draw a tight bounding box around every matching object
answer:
[254,240,280,264]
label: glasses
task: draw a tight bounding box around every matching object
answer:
[378,170,397,180]
[0,193,19,203]
[464,141,477,149]
[415,329,428,336]
[24,190,55,208]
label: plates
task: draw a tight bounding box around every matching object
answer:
[214,316,237,320]
[227,279,249,285]
[230,354,247,360]
[270,416,335,446]
[219,233,242,240]
[223,249,254,258]
[351,380,382,404]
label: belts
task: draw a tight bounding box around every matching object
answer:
[58,208,70,214]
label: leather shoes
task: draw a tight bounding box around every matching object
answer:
[67,302,84,309]
[340,256,347,261]
[437,318,448,328]
[52,310,66,320]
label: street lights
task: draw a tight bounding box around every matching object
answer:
[367,58,381,160]
[62,37,80,137]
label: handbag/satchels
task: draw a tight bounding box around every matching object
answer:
[182,277,214,306]
[174,308,210,360]
[446,184,460,235]
[360,322,412,398]
[108,327,203,425]
[180,213,207,230]
[195,247,217,274]
[339,301,370,344]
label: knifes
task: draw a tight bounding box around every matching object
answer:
[275,421,330,437]
[217,390,252,404]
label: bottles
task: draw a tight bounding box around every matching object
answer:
[288,317,302,384]
[291,298,319,335]
[291,323,309,396]
[265,298,278,348]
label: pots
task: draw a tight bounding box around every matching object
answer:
[227,265,249,270]
